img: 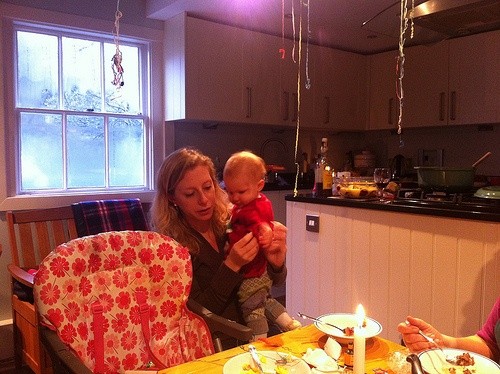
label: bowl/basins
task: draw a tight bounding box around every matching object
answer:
[314,312,383,345]
[333,177,378,199]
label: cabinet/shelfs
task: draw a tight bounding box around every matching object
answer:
[163,11,500,134]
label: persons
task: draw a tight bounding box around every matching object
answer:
[150,149,287,352]
[222,151,302,343]
[398,295,500,365]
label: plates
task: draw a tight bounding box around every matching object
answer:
[222,351,310,374]
[473,186,500,199]
[417,348,500,374]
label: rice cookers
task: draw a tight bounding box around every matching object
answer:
[353,151,377,167]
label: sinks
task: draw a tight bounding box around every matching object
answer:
[268,170,313,190]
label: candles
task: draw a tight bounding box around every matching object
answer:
[353,304,365,374]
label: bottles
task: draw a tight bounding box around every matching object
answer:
[316,138,332,200]
[344,341,354,367]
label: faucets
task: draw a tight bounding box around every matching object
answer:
[260,138,288,157]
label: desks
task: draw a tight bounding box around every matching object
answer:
[157,323,413,374]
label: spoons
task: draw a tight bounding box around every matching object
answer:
[297,312,345,334]
[405,320,463,366]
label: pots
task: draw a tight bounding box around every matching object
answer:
[413,166,475,194]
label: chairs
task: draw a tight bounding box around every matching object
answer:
[32,230,253,374]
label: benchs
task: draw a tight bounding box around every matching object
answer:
[5,202,151,374]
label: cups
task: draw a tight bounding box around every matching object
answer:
[332,171,361,177]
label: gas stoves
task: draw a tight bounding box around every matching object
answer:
[387,188,500,222]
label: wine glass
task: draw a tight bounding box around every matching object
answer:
[374,168,390,204]
